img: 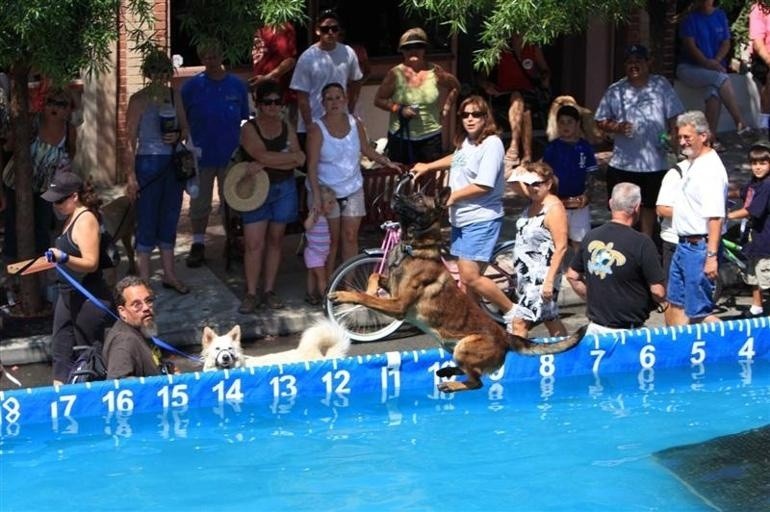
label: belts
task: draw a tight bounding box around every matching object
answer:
[679,236,702,243]
[593,321,642,329]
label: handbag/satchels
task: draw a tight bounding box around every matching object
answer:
[389,136,413,164]
[170,150,196,180]
[99,223,120,269]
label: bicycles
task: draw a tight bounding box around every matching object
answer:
[321,172,561,342]
[708,235,751,307]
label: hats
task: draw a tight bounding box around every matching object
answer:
[400,28,426,48]
[40,172,83,202]
[519,171,546,184]
[223,162,270,212]
[624,44,647,59]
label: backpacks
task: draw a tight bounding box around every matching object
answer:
[67,348,106,383]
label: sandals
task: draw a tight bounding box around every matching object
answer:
[304,292,319,305]
[713,140,727,153]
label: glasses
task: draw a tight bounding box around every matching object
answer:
[45,98,68,109]
[124,299,154,311]
[55,193,73,204]
[460,112,487,117]
[259,99,283,105]
[319,26,338,32]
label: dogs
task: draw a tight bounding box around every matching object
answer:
[326,186,589,393]
[198,316,352,371]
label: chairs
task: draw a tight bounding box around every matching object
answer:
[477,65,547,129]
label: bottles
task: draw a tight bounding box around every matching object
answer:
[626,111,635,138]
[158,98,177,144]
[410,100,419,120]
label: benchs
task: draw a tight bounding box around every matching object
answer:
[222,164,446,272]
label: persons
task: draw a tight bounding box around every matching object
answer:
[0,0,770,377]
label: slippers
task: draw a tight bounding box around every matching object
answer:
[163,280,189,294]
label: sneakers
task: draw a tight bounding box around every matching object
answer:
[261,291,283,309]
[239,294,261,314]
[224,238,244,263]
[186,243,205,268]
[503,302,518,334]
[733,311,764,320]
